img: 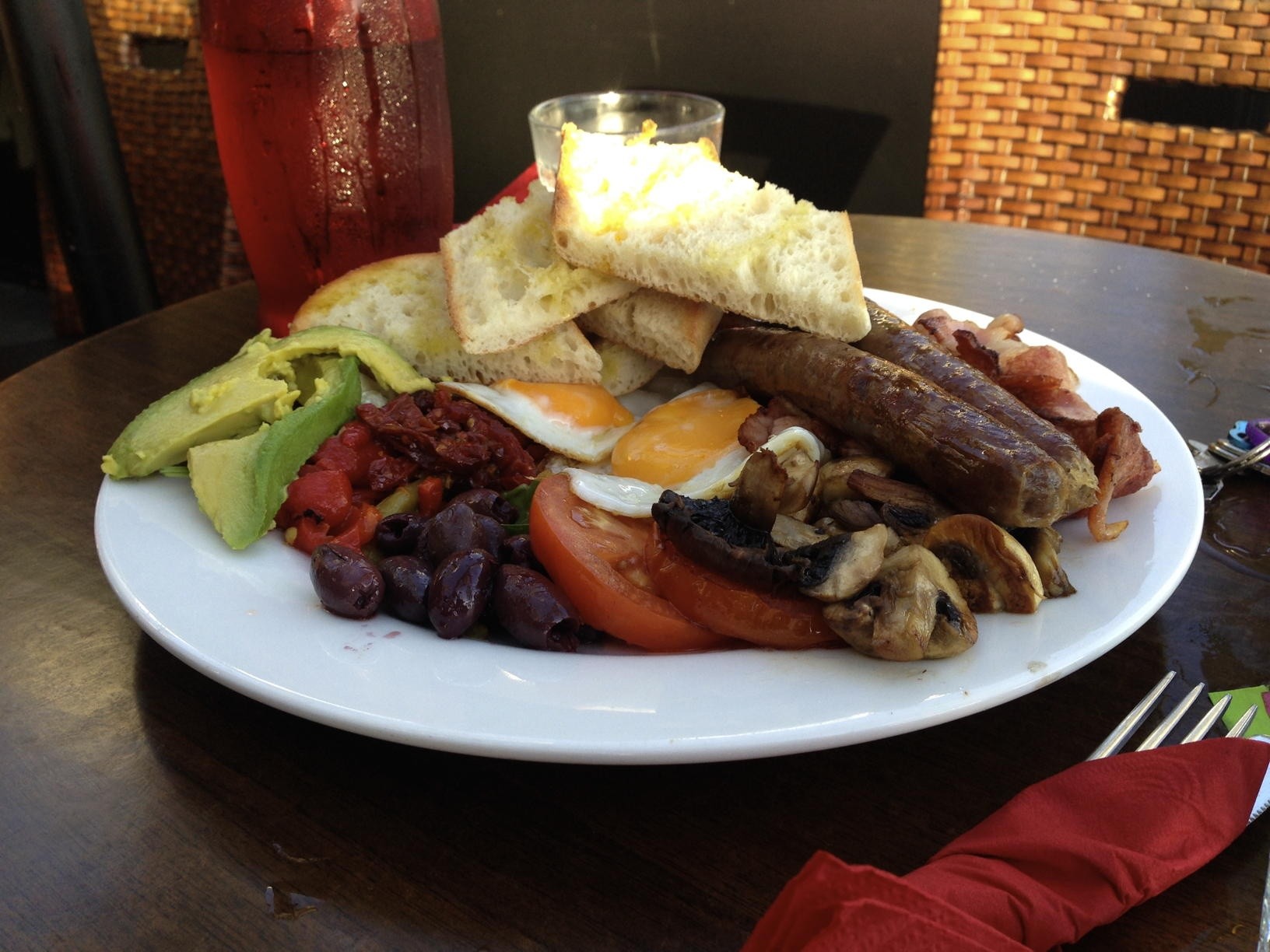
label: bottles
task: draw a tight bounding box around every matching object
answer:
[190,3,456,355]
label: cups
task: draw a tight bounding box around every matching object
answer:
[525,86,727,193]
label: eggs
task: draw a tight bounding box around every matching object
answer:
[437,379,760,516]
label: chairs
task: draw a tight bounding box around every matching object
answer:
[926,0,1270,276]
[35,1,256,345]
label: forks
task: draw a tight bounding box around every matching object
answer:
[1079,672,1257,763]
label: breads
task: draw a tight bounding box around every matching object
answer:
[287,121,874,400]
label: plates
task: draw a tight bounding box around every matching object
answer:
[86,278,1214,763]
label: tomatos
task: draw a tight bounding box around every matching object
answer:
[529,472,840,653]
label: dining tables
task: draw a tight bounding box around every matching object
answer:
[0,216,1270,950]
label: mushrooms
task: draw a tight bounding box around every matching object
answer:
[770,516,1045,663]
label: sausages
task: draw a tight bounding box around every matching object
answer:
[698,289,1101,530]
